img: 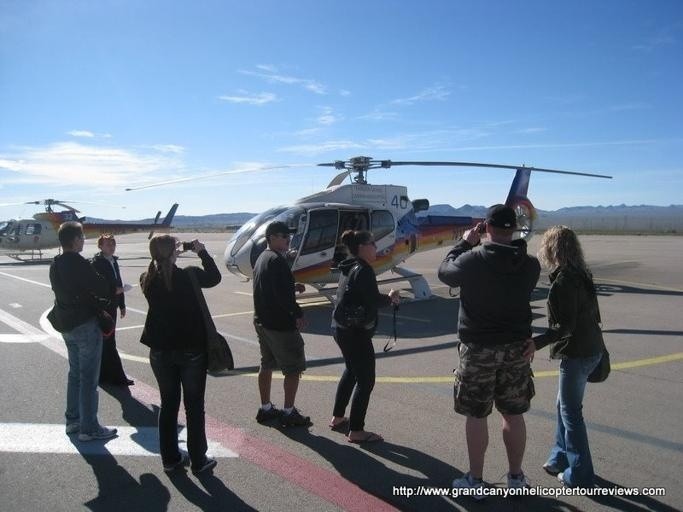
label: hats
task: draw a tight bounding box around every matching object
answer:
[487,204,516,227]
[266,222,297,234]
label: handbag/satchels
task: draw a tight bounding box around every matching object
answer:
[587,350,610,383]
[205,332,233,371]
[333,265,378,330]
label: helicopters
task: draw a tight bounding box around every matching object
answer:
[124,157,613,313]
[0,199,180,266]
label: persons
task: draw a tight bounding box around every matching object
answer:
[47,222,117,441]
[439,204,540,502]
[329,230,400,443]
[89,233,134,385]
[251,222,310,428]
[139,233,221,475]
[522,225,604,492]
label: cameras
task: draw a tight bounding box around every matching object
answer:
[183,242,194,251]
[479,223,486,232]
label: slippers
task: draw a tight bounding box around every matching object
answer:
[349,432,384,442]
[329,417,349,427]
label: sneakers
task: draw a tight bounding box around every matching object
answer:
[508,473,529,487]
[165,453,216,473]
[543,463,568,487]
[452,472,486,500]
[256,406,310,424]
[66,422,117,442]
